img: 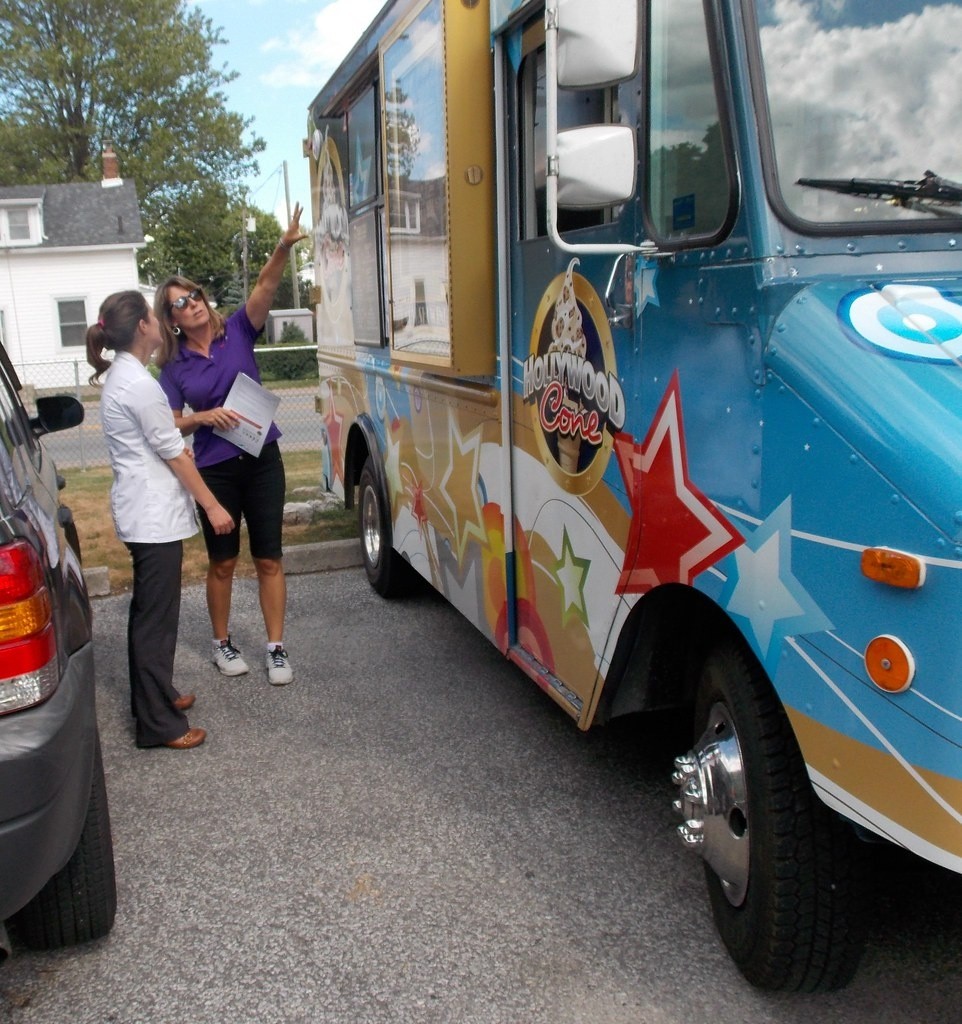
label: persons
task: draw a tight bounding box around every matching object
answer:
[85,290,235,749]
[154,201,308,684]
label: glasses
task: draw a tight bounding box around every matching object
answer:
[168,290,204,314]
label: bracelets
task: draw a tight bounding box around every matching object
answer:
[278,238,290,251]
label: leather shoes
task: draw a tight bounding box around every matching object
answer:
[158,726,207,750]
[171,693,196,709]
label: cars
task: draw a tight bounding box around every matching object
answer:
[0,340,116,964]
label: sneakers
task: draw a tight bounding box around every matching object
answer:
[263,644,294,685]
[211,635,250,677]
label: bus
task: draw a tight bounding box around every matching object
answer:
[303,0,962,993]
